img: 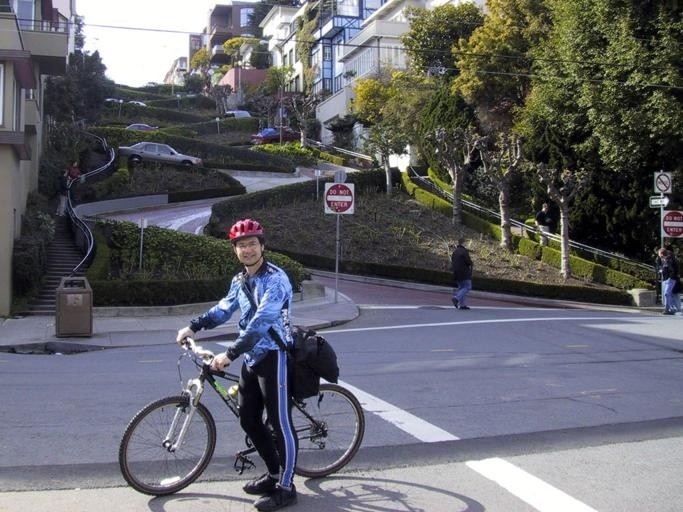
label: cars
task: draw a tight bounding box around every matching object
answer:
[251,125,302,147]
[122,122,158,133]
[224,110,251,119]
[117,141,202,170]
[127,101,147,107]
[106,98,123,104]
[144,81,158,89]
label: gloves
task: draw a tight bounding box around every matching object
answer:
[175,326,197,348]
[209,350,231,371]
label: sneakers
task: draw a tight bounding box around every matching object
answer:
[451,296,459,309]
[244,471,283,495]
[459,305,471,311]
[251,480,299,512]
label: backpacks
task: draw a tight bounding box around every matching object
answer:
[285,321,341,401]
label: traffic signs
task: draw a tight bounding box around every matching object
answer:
[648,196,670,209]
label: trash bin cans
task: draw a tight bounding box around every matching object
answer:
[55,277,94,337]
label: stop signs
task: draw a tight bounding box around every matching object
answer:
[660,210,682,239]
[322,179,354,215]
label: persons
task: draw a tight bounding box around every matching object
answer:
[658,245,681,315]
[55,169,69,217]
[451,238,472,309]
[535,202,551,246]
[176,219,298,512]
[69,161,80,179]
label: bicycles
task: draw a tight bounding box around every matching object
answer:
[115,332,366,496]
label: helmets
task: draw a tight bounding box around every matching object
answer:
[228,218,265,242]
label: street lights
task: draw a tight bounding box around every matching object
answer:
[259,40,286,146]
